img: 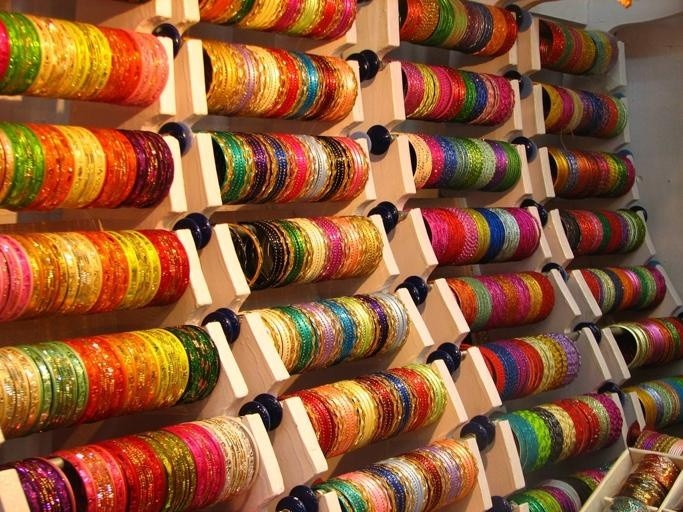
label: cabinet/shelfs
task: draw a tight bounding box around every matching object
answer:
[173,0,494,511]
[354,0,630,499]
[1,1,285,511]
[516,10,681,456]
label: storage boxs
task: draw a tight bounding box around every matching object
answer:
[580,447,682,510]
